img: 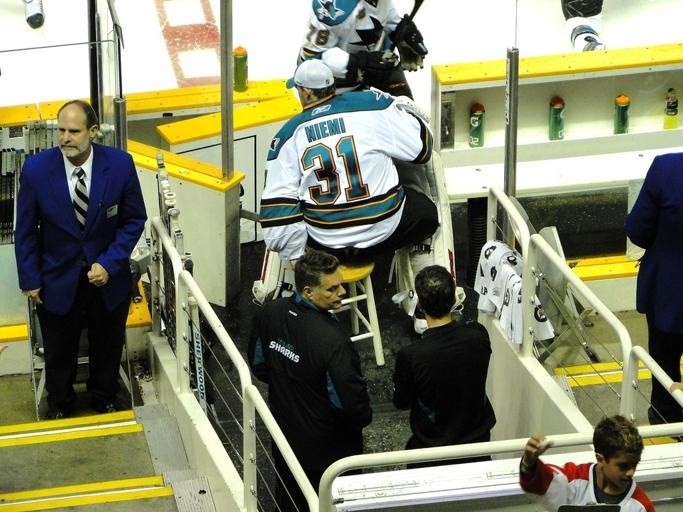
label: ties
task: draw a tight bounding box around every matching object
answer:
[71,168,88,233]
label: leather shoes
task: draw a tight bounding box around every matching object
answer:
[47,396,77,419]
[91,397,120,413]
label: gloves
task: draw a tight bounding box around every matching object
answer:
[346,51,399,83]
[388,15,428,72]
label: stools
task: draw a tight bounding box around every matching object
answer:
[328,260,386,367]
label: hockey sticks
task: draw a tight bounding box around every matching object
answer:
[389,0,425,52]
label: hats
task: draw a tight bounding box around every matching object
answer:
[286,60,334,89]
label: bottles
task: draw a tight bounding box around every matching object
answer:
[613,94,630,135]
[467,102,486,149]
[661,87,680,131]
[232,46,248,93]
[547,96,565,142]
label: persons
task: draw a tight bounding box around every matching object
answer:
[257,57,439,305]
[245,248,374,512]
[391,263,498,468]
[619,152,683,426]
[11,99,149,416]
[292,0,426,106]
[518,412,658,512]
